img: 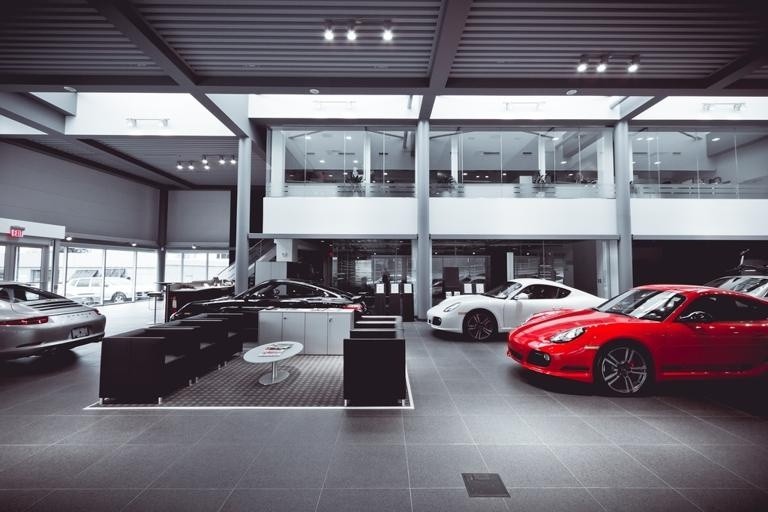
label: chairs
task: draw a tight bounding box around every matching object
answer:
[99,313,244,404]
[343,315,406,407]
[0,288,10,302]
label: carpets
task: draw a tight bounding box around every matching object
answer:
[91,354,410,407]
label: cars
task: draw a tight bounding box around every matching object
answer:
[704,265,768,304]
[58,277,153,304]
[0,281,107,361]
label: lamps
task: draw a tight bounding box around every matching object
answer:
[176,155,236,169]
[577,53,640,72]
[323,18,393,40]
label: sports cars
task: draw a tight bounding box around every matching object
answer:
[507,284,768,397]
[422,273,616,344]
[168,278,369,323]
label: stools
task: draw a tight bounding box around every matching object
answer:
[147,292,163,324]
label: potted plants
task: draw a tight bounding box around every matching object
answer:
[435,175,456,197]
[533,172,551,197]
[345,174,363,197]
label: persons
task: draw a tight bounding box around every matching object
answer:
[356,277,373,296]
[381,267,392,294]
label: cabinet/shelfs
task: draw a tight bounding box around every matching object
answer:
[258,308,354,355]
